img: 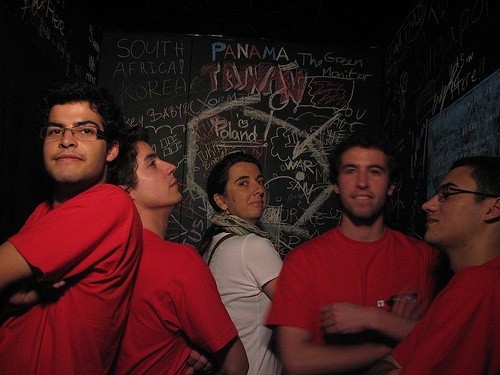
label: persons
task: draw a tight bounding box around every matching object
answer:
[199,152,284,375]
[391,156,500,375]
[105,122,248,375]
[265,128,435,375]
[0,77,143,375]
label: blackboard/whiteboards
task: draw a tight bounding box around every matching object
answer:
[377,0,500,245]
[92,15,419,255]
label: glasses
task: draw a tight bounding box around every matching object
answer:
[429,185,498,202]
[38,124,106,142]
